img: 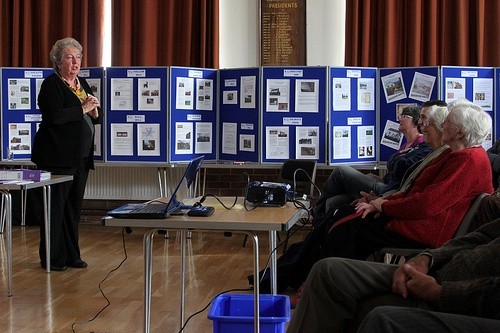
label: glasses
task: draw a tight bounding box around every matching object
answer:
[396,115,413,120]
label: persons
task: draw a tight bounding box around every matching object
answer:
[31,38,102,271]
[247,99,494,310]
[286,218,500,333]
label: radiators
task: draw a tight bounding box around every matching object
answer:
[82,164,192,200]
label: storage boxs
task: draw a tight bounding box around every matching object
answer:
[207,294,290,333]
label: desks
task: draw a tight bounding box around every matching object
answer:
[0,175,74,297]
[101,196,310,333]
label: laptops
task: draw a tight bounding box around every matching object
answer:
[106,155,204,218]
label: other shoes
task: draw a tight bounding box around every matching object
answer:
[41,263,67,271]
[68,259,88,268]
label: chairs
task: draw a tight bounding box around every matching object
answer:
[243,159,318,254]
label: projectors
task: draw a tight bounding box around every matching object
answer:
[245,180,291,206]
